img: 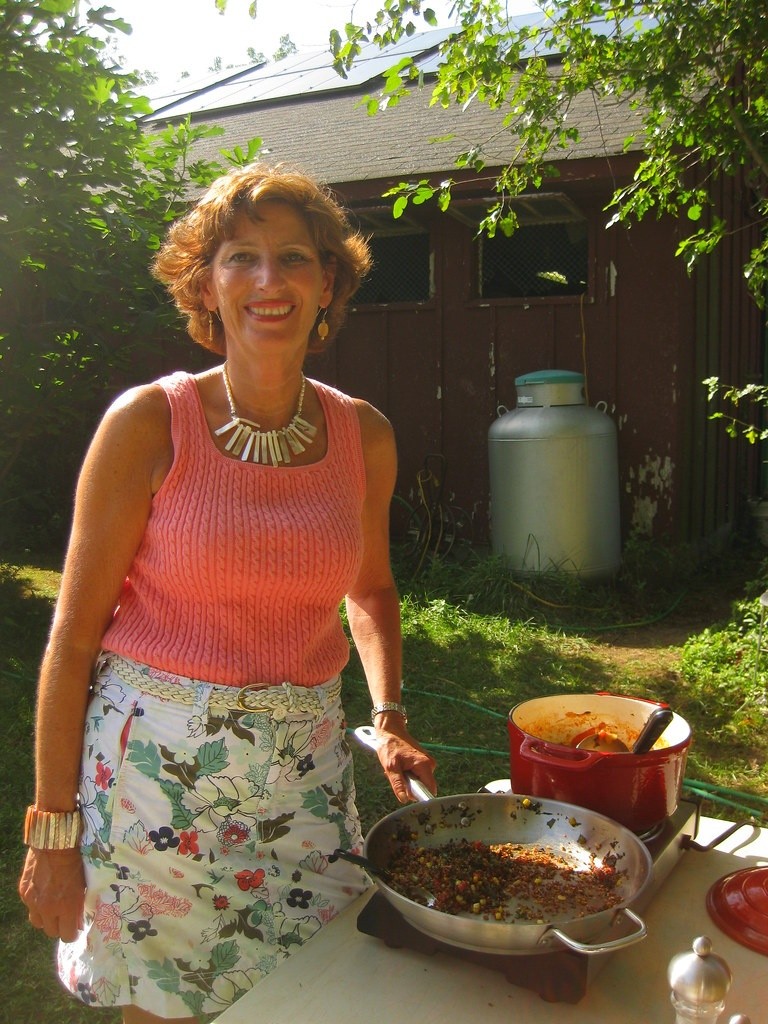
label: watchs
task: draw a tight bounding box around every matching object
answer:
[370,701,409,725]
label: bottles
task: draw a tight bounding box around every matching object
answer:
[667,936,732,1024]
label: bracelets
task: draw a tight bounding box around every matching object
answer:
[22,804,86,854]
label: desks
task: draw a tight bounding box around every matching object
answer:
[210,778,768,1023]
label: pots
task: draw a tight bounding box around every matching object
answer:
[507,693,691,834]
[355,724,653,954]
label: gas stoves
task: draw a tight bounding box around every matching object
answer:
[356,777,700,1003]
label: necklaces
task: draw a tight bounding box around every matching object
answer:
[213,362,319,465]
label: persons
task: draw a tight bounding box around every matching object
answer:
[17,160,438,1023]
